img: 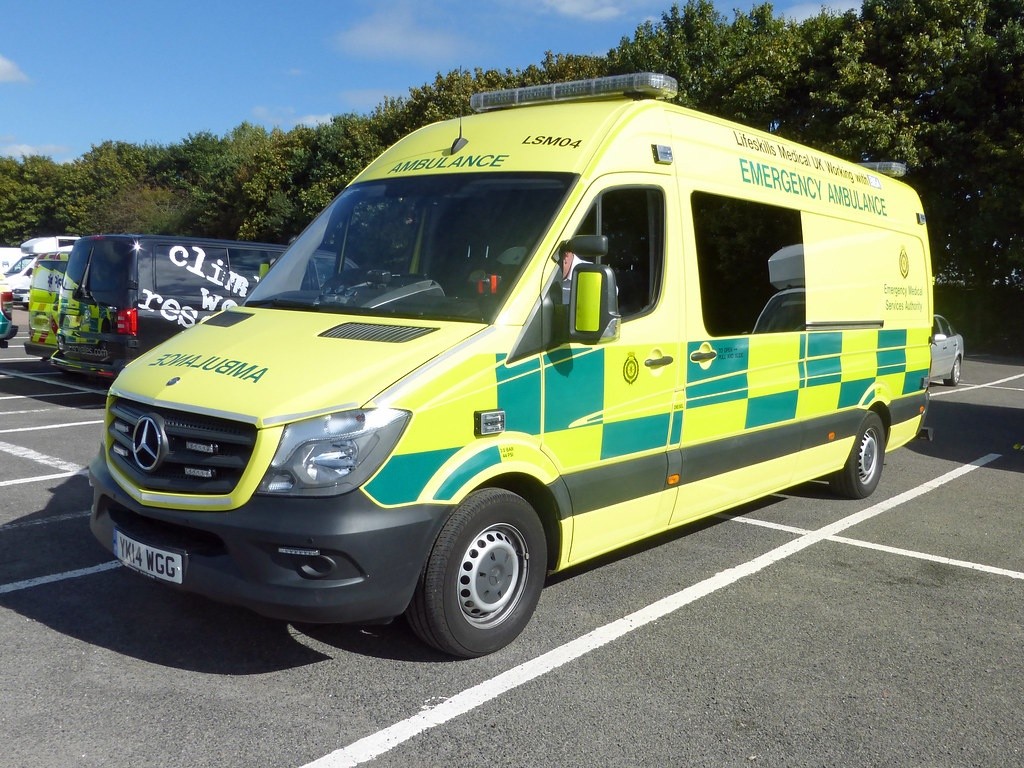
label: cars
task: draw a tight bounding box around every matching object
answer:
[0,237,82,310]
[933,314,966,386]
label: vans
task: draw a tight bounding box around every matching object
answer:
[49,233,361,383]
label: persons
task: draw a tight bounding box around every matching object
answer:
[562,249,619,307]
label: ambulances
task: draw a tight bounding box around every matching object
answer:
[21,250,79,359]
[89,72,934,659]
